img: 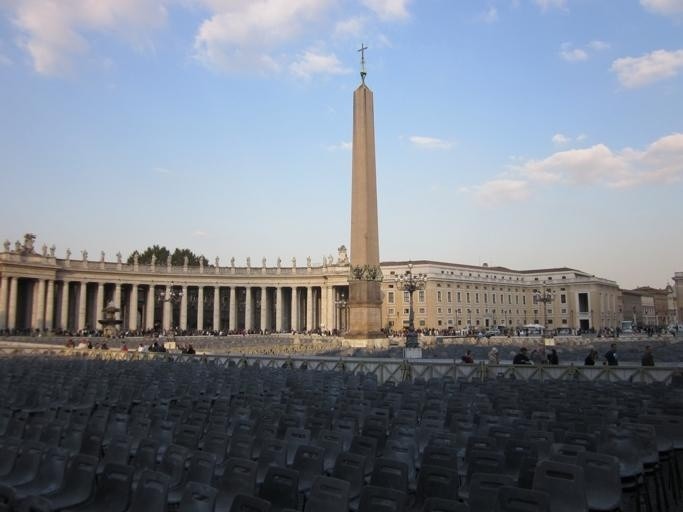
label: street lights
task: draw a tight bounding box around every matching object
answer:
[394,260,427,360]
[534,281,555,345]
[157,280,184,342]
[332,293,348,333]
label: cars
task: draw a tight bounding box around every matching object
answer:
[453,324,510,337]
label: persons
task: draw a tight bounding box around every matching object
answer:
[0,319,682,372]
